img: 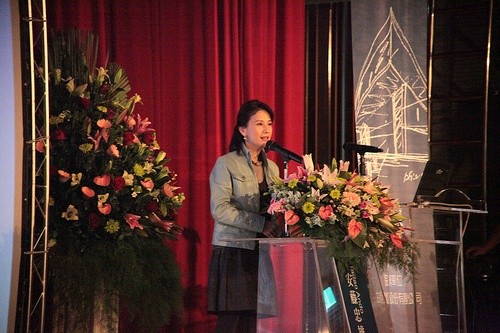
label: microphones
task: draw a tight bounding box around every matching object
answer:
[266,140,304,164]
[343,141,383,153]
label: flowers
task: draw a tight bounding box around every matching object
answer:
[33,27,185,331]
[263,153,421,277]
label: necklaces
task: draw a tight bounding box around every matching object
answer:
[252,160,263,166]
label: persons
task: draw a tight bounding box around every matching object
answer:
[205,99,280,333]
